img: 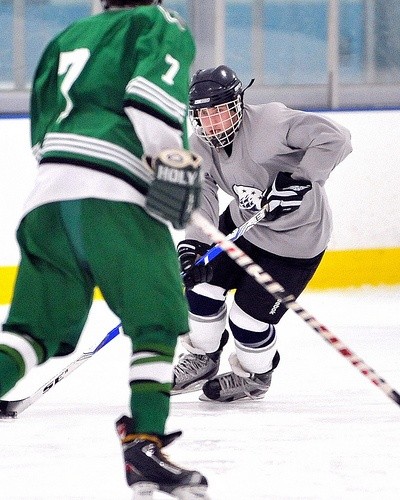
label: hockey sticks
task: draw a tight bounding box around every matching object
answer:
[1,201,280,418]
[187,210,400,404]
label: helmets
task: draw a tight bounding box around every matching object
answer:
[188,65,243,150]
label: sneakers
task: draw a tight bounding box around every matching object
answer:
[170,328,229,395]
[116,415,208,500]
[198,351,280,404]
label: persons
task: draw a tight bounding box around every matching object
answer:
[0,0,218,500]
[170,65,355,408]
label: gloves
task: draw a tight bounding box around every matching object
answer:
[261,170,312,220]
[145,149,203,231]
[177,239,219,288]
